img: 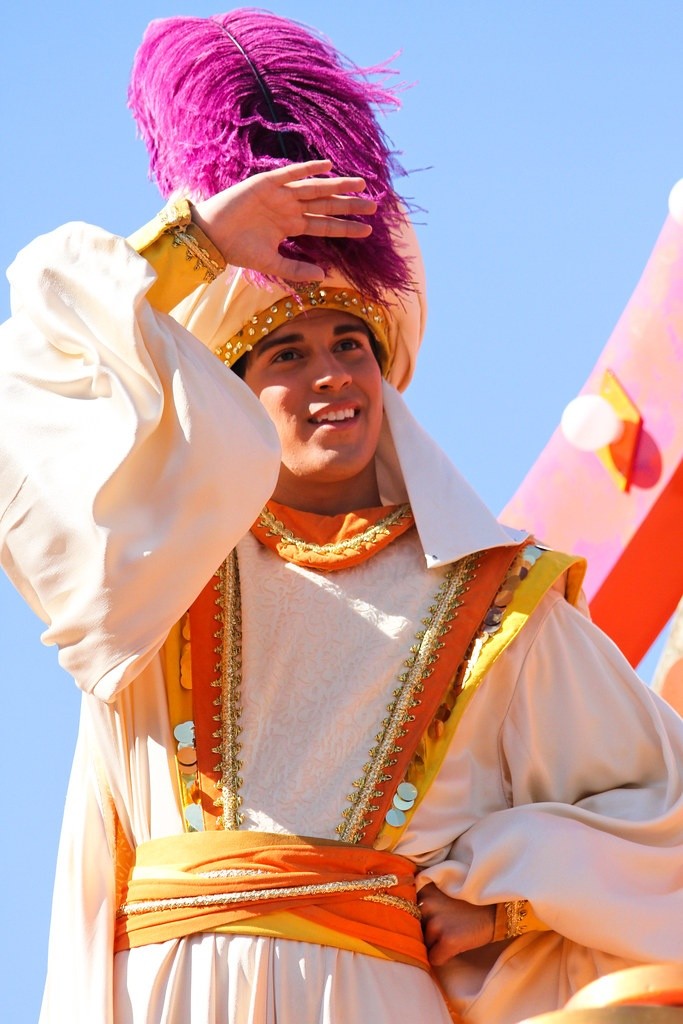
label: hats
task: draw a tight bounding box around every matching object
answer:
[127,8,424,391]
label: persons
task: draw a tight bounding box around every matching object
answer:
[0,9,683,1024]
